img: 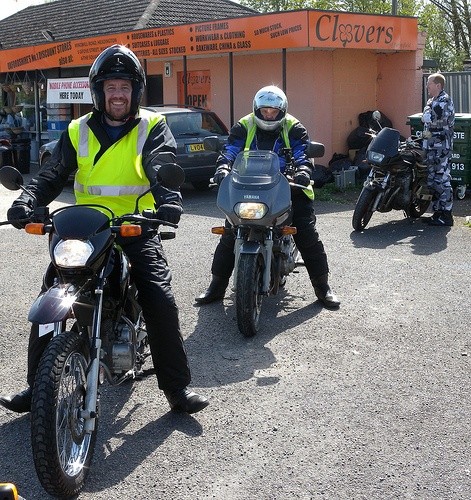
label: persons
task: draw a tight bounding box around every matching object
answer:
[194,85,341,309]
[0,43,210,415]
[421,73,456,226]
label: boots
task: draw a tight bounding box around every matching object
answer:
[194,276,229,302]
[423,208,443,223]
[429,208,454,226]
[311,273,341,307]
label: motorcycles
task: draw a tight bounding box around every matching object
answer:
[0,162,185,500]
[202,135,325,338]
[351,110,433,232]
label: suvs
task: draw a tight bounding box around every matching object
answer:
[38,104,230,195]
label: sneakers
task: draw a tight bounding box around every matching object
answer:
[166,387,210,415]
[0,386,34,412]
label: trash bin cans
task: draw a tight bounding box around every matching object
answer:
[0,139,12,169]
[11,137,30,175]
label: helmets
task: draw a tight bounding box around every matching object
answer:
[253,85,288,131]
[89,44,145,116]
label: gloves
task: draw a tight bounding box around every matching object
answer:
[292,165,312,190]
[7,199,33,229]
[156,203,183,224]
[214,168,229,185]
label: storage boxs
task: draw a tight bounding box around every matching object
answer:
[46,103,72,142]
